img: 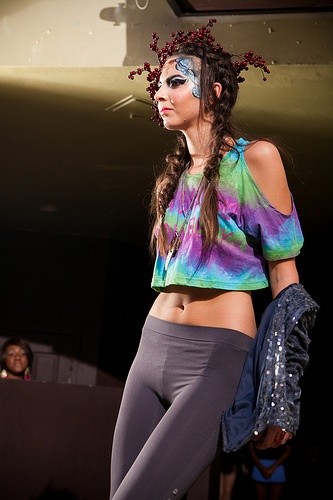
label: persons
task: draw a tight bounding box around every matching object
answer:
[109,16,304,499]
[218,451,249,500]
[0,337,35,380]
[249,439,291,500]
